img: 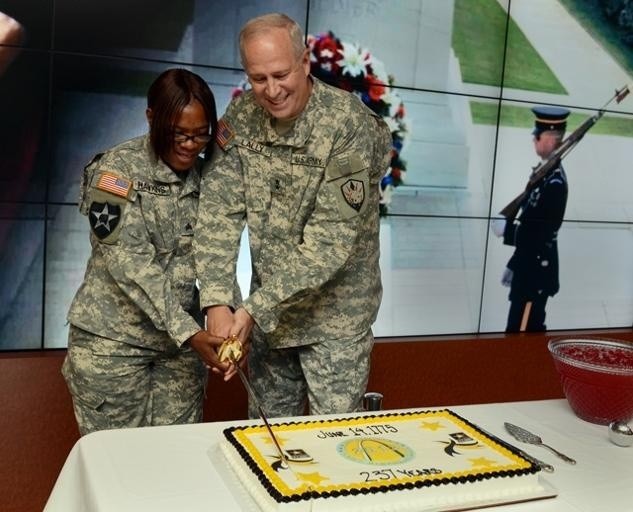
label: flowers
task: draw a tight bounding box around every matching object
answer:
[225,28,409,223]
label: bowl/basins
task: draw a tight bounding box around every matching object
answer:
[548,334,633,427]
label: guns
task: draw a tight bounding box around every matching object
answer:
[499,84,630,224]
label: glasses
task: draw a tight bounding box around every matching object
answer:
[534,133,562,141]
[167,128,213,145]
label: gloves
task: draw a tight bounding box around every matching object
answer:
[491,214,508,237]
[500,267,515,288]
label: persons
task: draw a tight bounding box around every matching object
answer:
[58,68,250,438]
[490,105,571,332]
[190,12,394,420]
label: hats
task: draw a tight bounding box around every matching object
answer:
[528,106,572,134]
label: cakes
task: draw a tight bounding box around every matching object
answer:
[219,408,543,512]
